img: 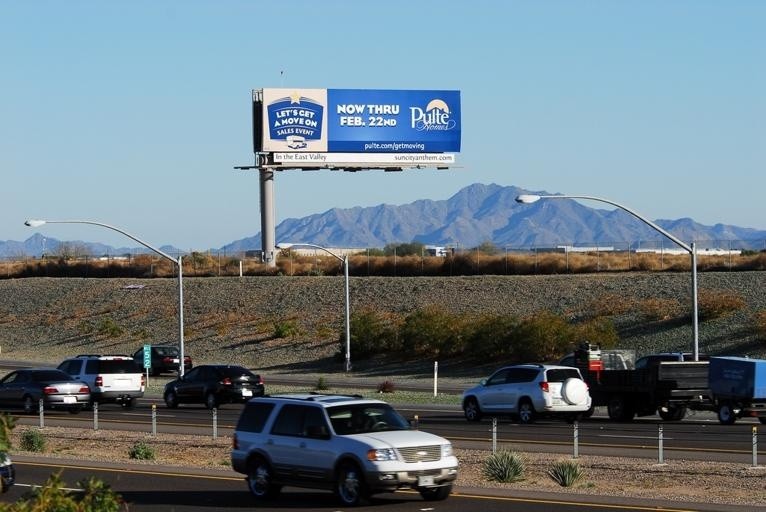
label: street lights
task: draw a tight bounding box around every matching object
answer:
[515,195,699,360]
[274,243,352,372]
[25,219,184,378]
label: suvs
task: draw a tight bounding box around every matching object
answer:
[559,342,765,424]
[462,364,593,425]
[231,391,460,506]
[57,353,145,412]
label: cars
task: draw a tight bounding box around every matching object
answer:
[1,367,91,415]
[130,345,193,375]
[164,365,265,410]
